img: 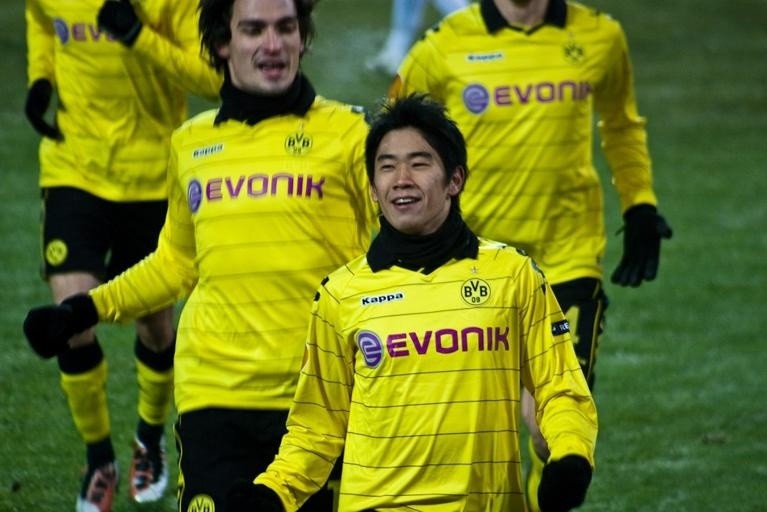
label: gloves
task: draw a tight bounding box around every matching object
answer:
[611,202,671,288]
[25,79,64,140]
[23,294,99,358]
[537,455,592,512]
[97,2,145,49]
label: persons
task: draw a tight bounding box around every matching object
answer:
[22,1,226,512]
[379,1,673,512]
[22,1,378,512]
[364,2,472,81]
[225,94,597,511]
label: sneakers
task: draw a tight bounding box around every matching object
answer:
[77,462,119,512]
[130,433,168,504]
